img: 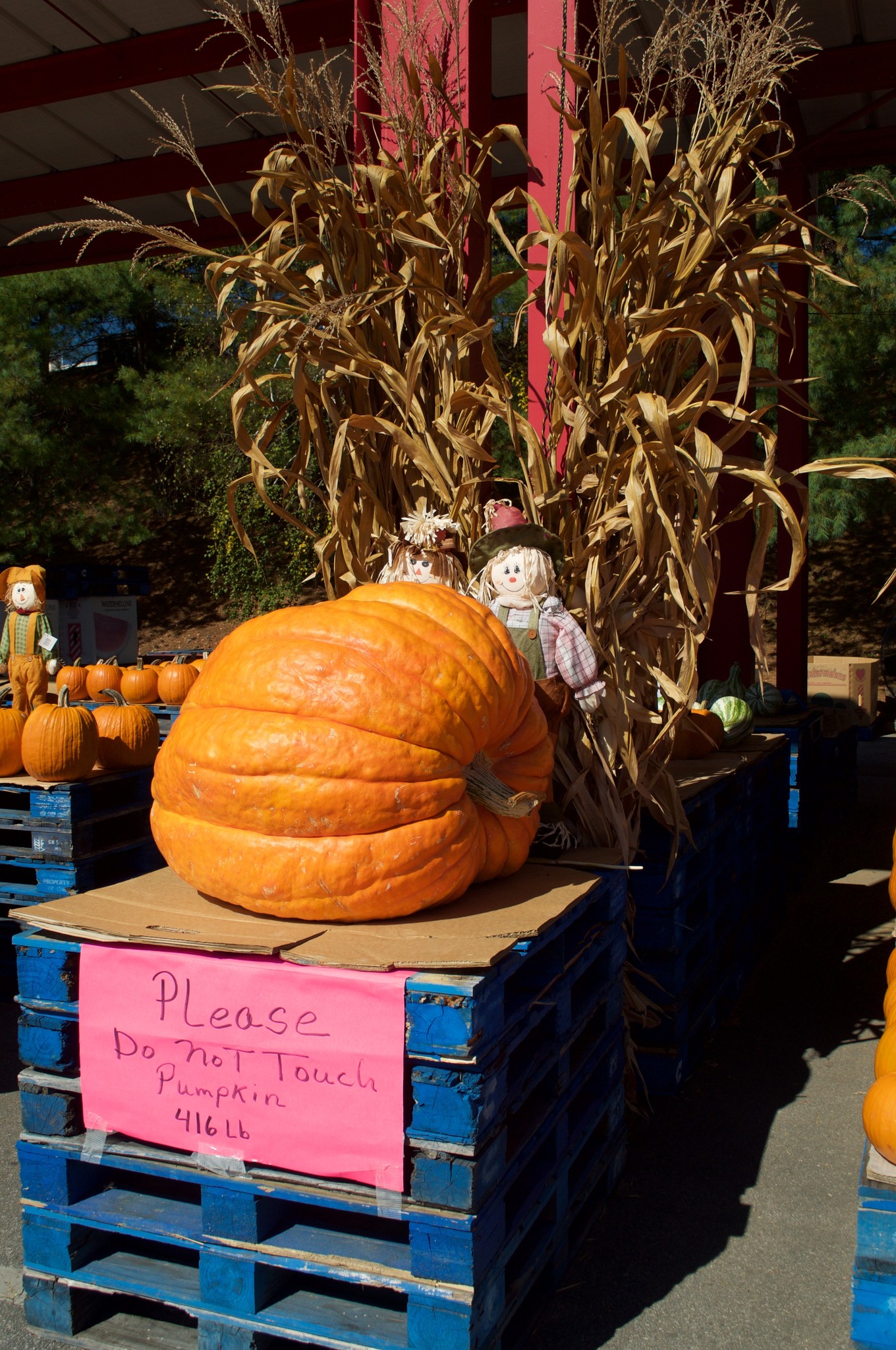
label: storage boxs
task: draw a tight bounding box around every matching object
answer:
[1,596,139,666]
[808,655,881,725]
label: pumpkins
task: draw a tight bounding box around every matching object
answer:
[122,660,158,704]
[55,657,89,700]
[190,652,208,673]
[86,656,122,704]
[148,580,556,921]
[90,689,160,768]
[84,655,183,676]
[20,684,98,782]
[1,706,26,778]
[156,653,199,704]
